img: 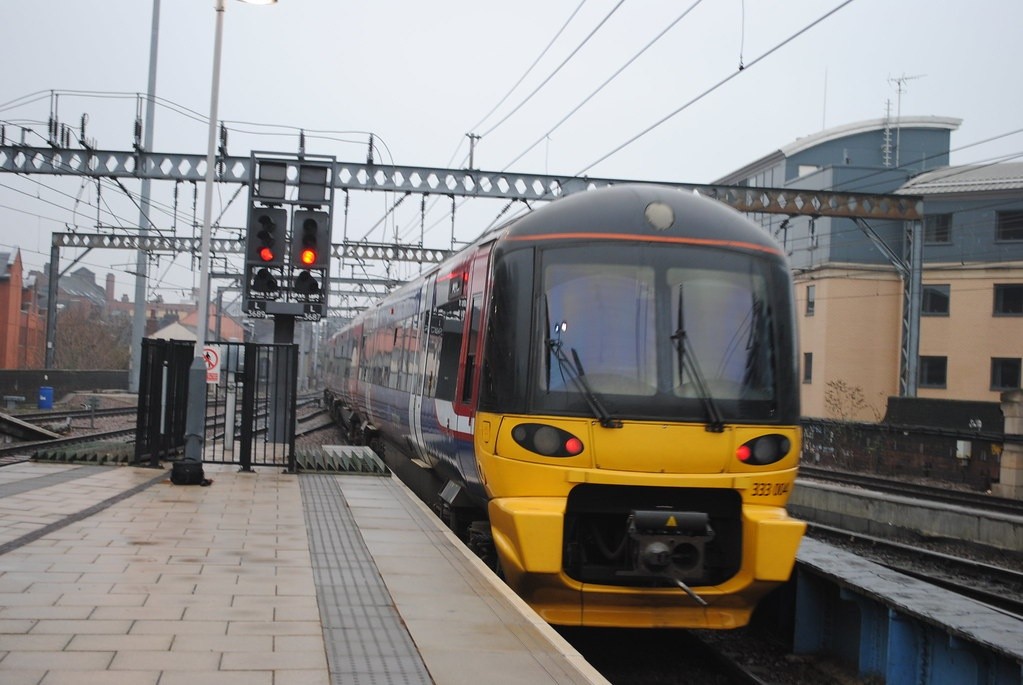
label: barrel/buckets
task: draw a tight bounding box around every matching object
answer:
[38,386,53,409]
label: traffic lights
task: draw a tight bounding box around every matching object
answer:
[258,214,274,262]
[300,219,317,264]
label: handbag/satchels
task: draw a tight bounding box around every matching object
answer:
[170,457,204,485]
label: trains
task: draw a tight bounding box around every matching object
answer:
[313,178,807,655]
